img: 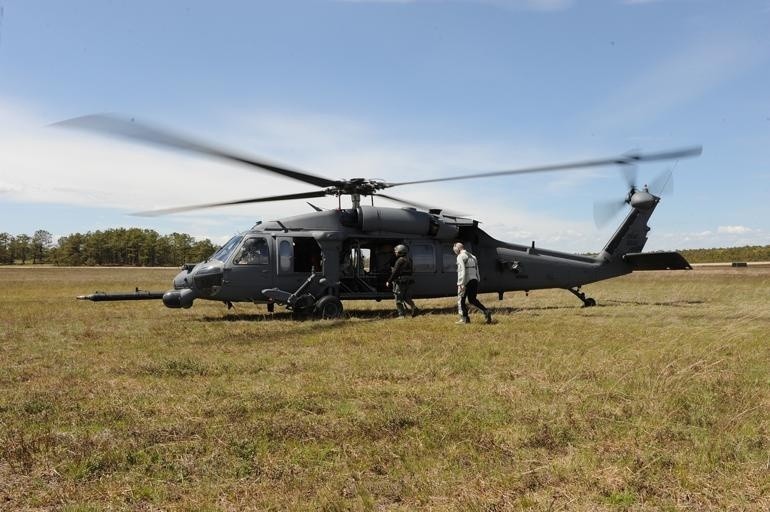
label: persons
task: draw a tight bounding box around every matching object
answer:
[386,244,418,317]
[453,242,492,324]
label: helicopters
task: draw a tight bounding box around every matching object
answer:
[43,111,703,321]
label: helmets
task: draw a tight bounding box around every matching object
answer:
[394,245,408,257]
[453,243,464,255]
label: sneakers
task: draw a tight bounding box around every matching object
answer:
[456,318,470,323]
[396,306,419,319]
[485,310,492,323]
